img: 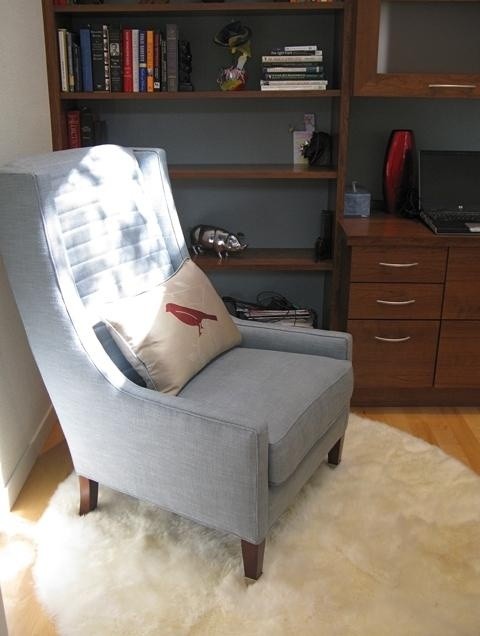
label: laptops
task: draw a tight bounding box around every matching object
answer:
[418,150,480,235]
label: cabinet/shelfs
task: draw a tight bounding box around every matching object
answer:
[41,0,355,333]
[325,1,480,407]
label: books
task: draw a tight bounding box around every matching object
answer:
[55,21,183,94]
[259,43,328,92]
[66,109,107,147]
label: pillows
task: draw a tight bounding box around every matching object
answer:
[98,258,242,395]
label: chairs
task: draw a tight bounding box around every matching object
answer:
[0,143,357,581]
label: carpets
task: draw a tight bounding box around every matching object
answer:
[32,412,480,636]
